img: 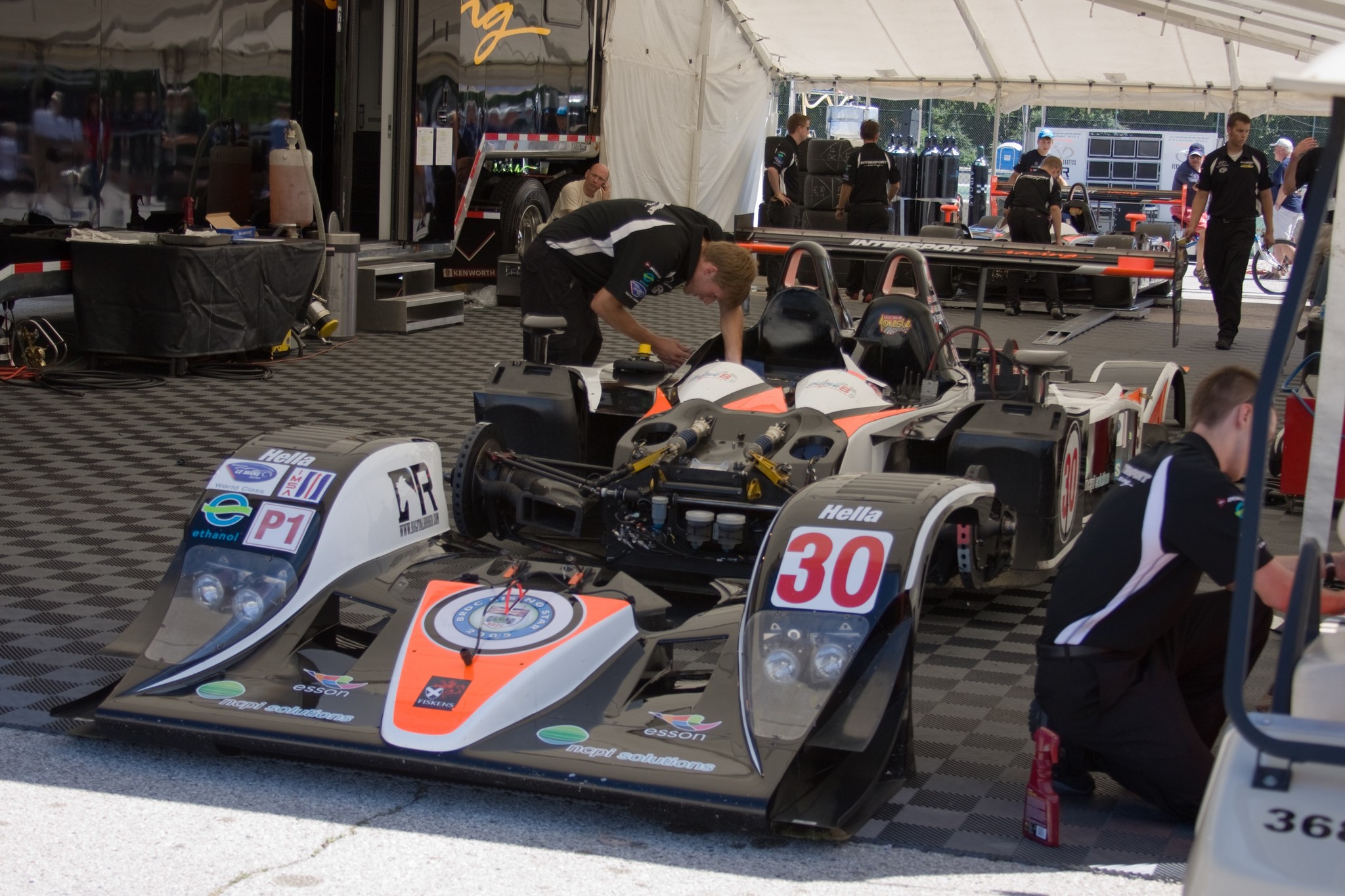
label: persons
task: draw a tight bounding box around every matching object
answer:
[521,198,757,369]
[835,119,903,302]
[0,87,212,211]
[531,165,609,241]
[1006,128,1067,187]
[1281,137,1338,316]
[1182,111,1275,349]
[765,114,810,299]
[1004,155,1068,321]
[1027,365,1345,826]
[1260,138,1303,280]
[1169,142,1211,285]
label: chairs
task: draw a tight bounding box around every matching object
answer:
[1063,200,1096,235]
[710,283,840,383]
[854,295,958,398]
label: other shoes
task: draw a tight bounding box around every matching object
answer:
[1259,273,1282,280]
[766,291,777,301]
[1028,711,1097,797]
[1215,335,1233,349]
[862,293,872,303]
[1194,268,1209,284]
[1004,299,1022,315]
[845,289,859,300]
[1200,283,1210,290]
[1051,302,1066,319]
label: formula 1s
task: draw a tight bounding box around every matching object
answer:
[43,240,1193,831]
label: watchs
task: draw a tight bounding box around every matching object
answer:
[836,206,844,211]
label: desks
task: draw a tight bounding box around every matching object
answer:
[70,231,322,372]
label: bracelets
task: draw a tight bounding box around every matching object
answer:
[774,193,781,198]
[1322,552,1335,590]
[1274,203,1281,209]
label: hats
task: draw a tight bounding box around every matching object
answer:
[1039,128,1054,140]
[1269,139,1293,150]
[1188,143,1205,158]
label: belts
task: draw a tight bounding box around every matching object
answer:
[1012,206,1036,211]
[1034,642,1109,659]
[1210,215,1255,223]
[852,202,883,205]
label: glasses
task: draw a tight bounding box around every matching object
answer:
[1272,146,1281,151]
[1055,166,1060,180]
[797,124,810,131]
[1189,154,1202,160]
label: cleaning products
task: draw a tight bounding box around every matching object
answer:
[1021,715,1062,847]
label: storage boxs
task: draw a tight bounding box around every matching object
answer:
[203,210,257,242]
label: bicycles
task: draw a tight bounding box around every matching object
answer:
[1169,210,1297,296]
